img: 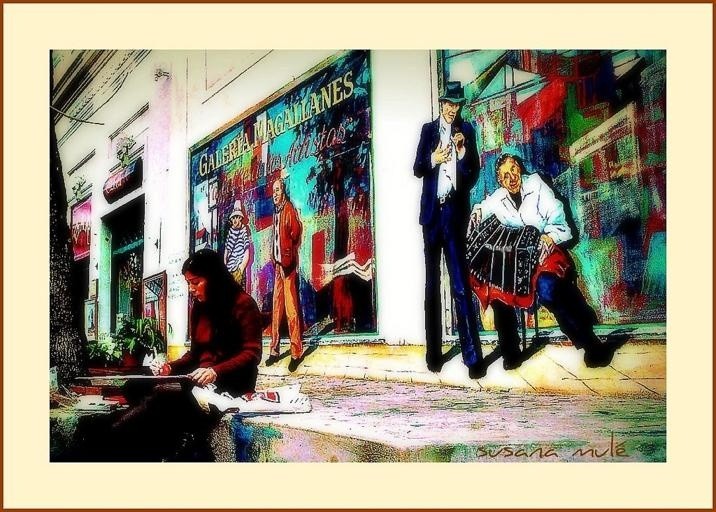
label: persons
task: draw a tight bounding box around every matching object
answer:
[223,200,250,289]
[413,80,486,378]
[265,179,304,372]
[112,249,262,462]
[468,153,606,369]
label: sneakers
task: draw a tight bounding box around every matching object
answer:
[266,355,276,365]
[288,356,303,372]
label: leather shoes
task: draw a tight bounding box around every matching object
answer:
[503,350,522,369]
[583,349,614,367]
[426,357,441,372]
[468,362,486,380]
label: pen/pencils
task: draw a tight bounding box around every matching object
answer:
[152,346,160,375]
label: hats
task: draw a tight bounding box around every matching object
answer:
[438,81,466,104]
[229,199,245,219]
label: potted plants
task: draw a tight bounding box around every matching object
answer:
[110,314,172,366]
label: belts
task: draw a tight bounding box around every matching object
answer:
[437,197,448,204]
